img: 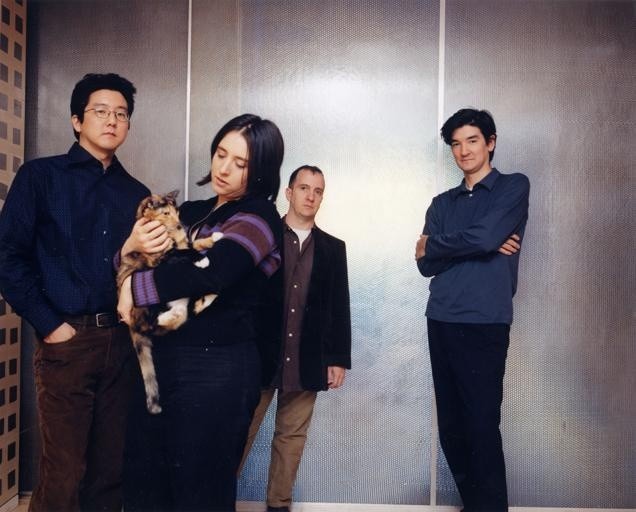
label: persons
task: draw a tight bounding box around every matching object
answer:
[0,73,153,511]
[236,163,353,511]
[414,108,532,511]
[114,113,288,511]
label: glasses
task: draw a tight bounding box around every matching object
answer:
[82,105,130,122]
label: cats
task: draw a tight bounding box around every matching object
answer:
[116,189,223,414]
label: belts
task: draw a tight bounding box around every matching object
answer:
[59,311,125,328]
